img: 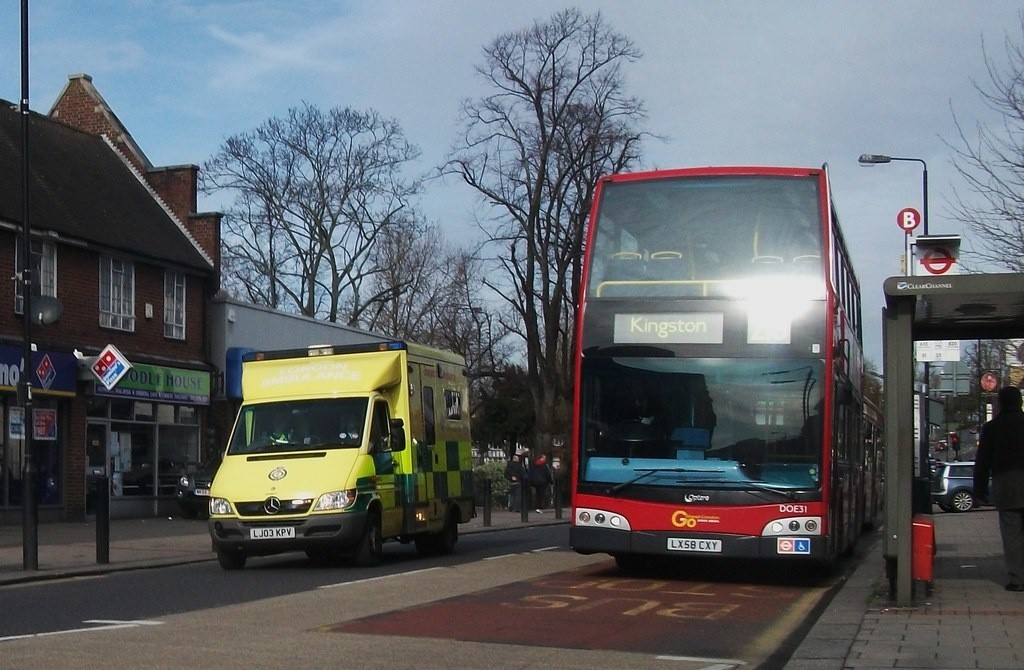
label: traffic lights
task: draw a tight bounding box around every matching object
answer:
[952,435,959,451]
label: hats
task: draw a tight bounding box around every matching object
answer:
[997,386,1023,403]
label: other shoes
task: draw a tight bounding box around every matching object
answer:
[509,507,521,512]
[1004,581,1024,591]
[536,507,542,513]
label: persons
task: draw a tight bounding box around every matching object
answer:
[974,386,1024,592]
[527,453,554,514]
[616,373,669,458]
[504,455,526,512]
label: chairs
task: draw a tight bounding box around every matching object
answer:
[600,246,825,300]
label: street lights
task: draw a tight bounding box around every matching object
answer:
[859,154,927,237]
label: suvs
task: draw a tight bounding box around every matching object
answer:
[931,461,1000,512]
[176,445,224,519]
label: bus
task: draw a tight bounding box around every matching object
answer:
[568,164,884,577]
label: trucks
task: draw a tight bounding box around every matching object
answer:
[43,431,153,506]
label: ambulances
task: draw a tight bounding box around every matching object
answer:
[207,339,475,575]
